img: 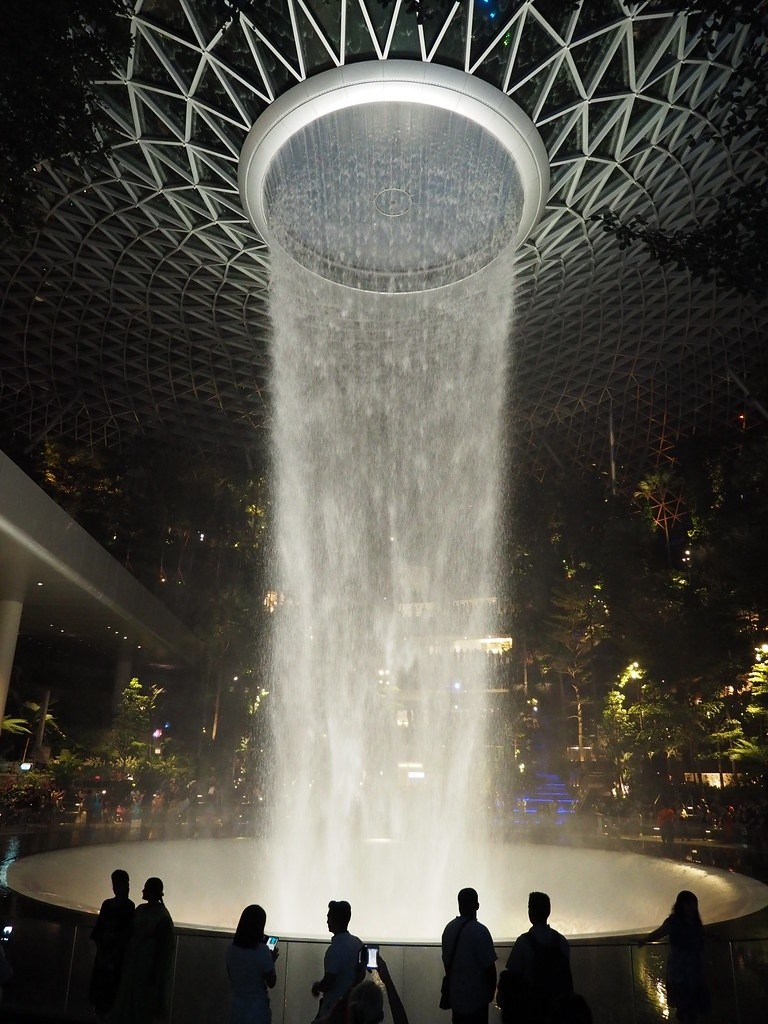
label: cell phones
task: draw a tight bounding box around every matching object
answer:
[267,936,279,954]
[367,945,379,969]
[0,926,12,941]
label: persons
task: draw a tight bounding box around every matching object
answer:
[89,870,176,1024]
[442,888,499,1023]
[225,906,279,1024]
[311,901,365,1024]
[327,950,408,1024]
[656,798,709,844]
[628,891,721,1024]
[497,892,572,1024]
